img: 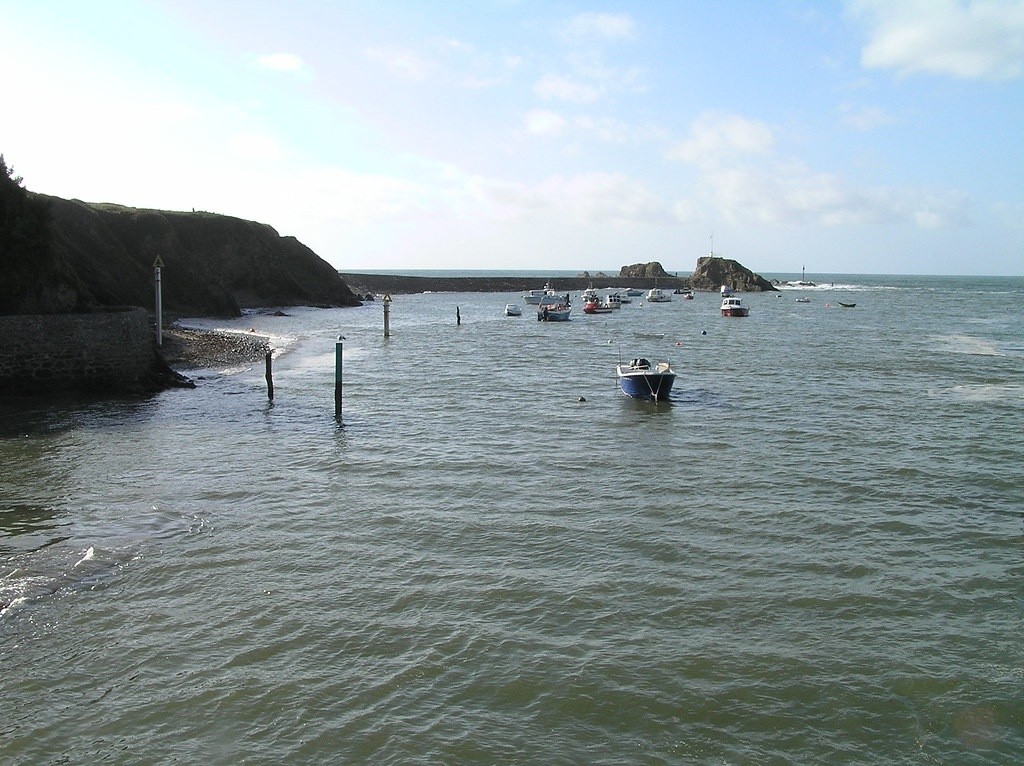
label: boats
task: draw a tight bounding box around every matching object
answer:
[606,292,622,308]
[538,293,571,321]
[646,288,671,302]
[627,290,644,296]
[796,297,810,302]
[839,302,856,308]
[582,289,599,301]
[674,289,691,294]
[505,304,521,316]
[721,284,734,297]
[684,293,693,299]
[583,301,612,314]
[622,295,633,303]
[521,286,555,304]
[720,295,747,316]
[787,265,816,287]
[616,347,677,401]
